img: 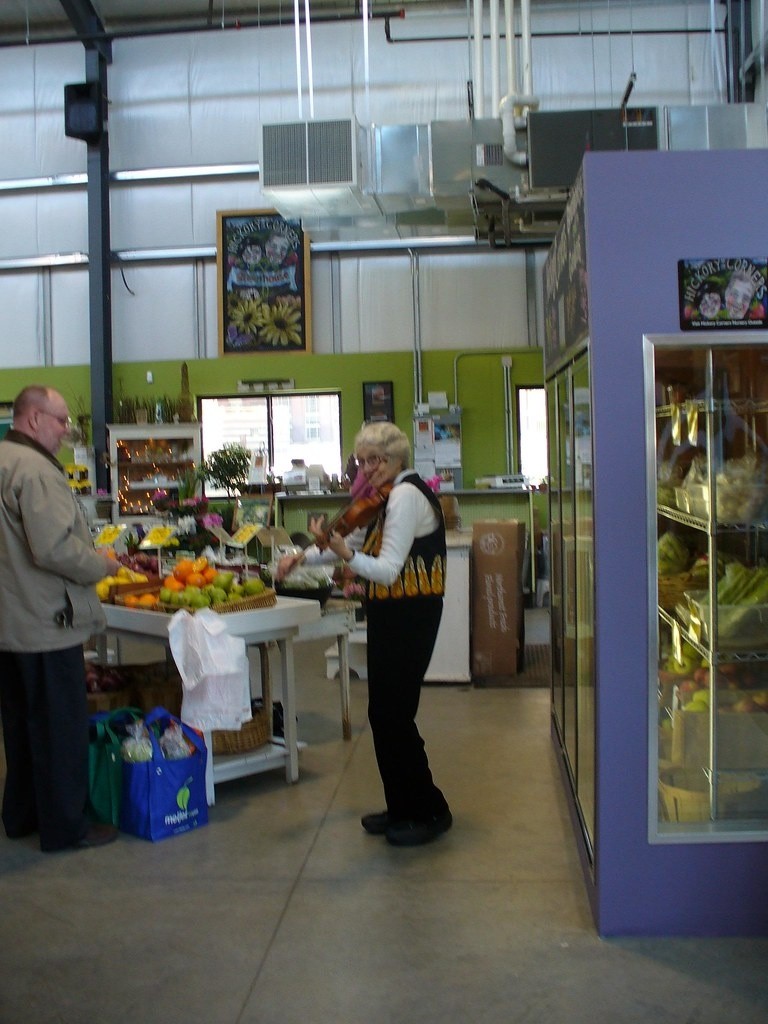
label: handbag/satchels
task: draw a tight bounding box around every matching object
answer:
[116,706,212,843]
[86,705,159,827]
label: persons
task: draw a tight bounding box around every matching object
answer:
[278,419,454,847]
[0,383,120,854]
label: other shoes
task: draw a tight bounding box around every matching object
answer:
[362,810,402,835]
[40,828,118,854]
[385,808,453,843]
[4,815,55,839]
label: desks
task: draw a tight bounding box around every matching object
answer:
[100,595,362,807]
[423,526,474,683]
[105,422,201,524]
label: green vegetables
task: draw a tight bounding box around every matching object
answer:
[283,576,333,589]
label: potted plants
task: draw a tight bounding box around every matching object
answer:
[124,531,139,557]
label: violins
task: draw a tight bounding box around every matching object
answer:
[314,482,393,552]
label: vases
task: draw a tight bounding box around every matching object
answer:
[199,504,208,514]
[73,487,91,496]
[156,502,166,512]
[95,497,111,518]
[68,470,88,480]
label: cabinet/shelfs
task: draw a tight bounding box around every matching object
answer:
[658,345,768,832]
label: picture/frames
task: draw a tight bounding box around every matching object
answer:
[216,208,313,357]
[363,380,395,424]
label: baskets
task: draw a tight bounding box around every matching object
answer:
[212,709,275,756]
[87,688,132,714]
[113,582,277,617]
[136,683,179,716]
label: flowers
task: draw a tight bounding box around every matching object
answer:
[97,487,107,497]
[65,462,88,476]
[66,478,92,488]
[180,493,209,507]
[151,488,164,500]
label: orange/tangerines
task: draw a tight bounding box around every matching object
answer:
[124,557,218,609]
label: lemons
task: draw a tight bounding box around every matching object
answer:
[96,565,148,600]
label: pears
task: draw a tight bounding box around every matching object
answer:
[161,573,265,607]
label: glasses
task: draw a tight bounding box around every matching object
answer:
[355,456,385,465]
[38,411,71,427]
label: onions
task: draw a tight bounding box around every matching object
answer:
[84,661,155,692]
[118,554,157,569]
